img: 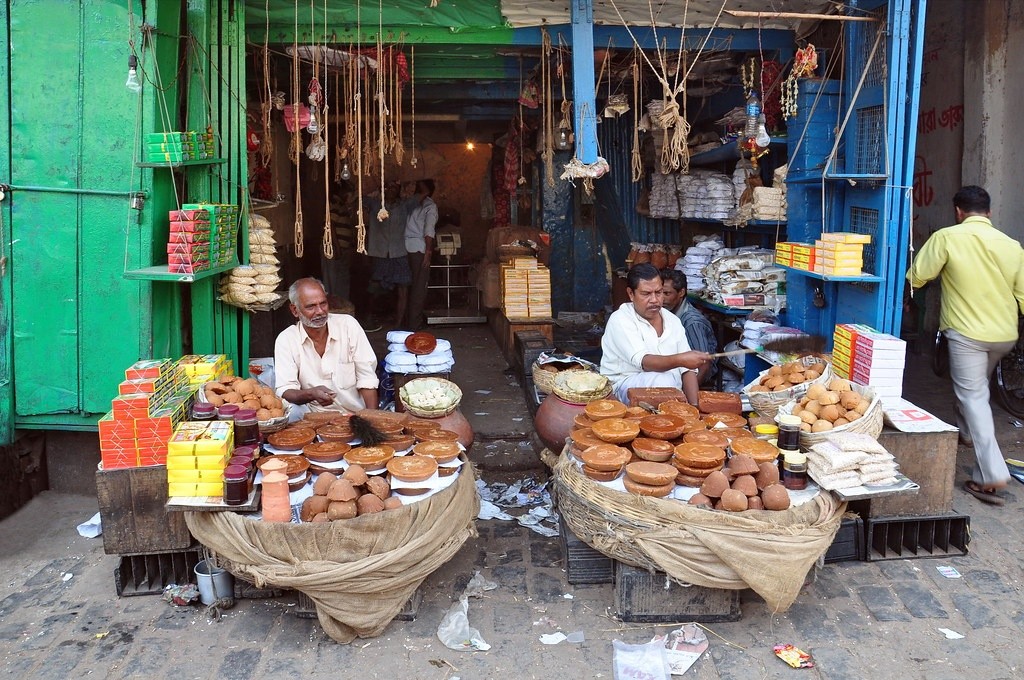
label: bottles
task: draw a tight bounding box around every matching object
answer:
[755,414,808,490]
[221,446,254,505]
[193,403,259,446]
[745,92,760,137]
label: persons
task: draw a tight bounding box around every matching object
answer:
[905,185,1024,505]
[275,277,380,418]
[600,263,715,406]
[658,267,719,387]
[321,179,438,333]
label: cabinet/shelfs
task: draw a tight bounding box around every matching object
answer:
[643,136,787,317]
[772,171,890,282]
[123,156,238,283]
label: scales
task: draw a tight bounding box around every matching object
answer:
[422,232,488,329]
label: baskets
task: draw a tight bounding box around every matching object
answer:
[532,352,574,396]
[400,377,462,418]
[551,370,612,404]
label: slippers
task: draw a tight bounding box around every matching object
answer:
[964,480,1005,504]
[959,434,974,447]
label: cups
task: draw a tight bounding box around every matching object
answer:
[260,458,288,478]
[261,471,292,522]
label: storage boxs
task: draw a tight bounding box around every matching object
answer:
[146,132,237,274]
[774,231,871,277]
[96,355,233,497]
[831,323,907,400]
[483,225,558,419]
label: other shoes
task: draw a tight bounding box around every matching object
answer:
[364,324,382,332]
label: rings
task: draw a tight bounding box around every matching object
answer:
[704,359,706,364]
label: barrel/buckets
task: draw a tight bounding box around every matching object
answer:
[194,557,235,607]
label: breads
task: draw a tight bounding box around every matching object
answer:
[749,360,824,394]
[567,386,790,513]
[203,376,460,523]
[404,332,437,354]
[793,379,872,433]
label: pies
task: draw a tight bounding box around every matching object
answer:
[408,388,451,407]
[566,373,608,392]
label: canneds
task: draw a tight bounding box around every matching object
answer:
[192,402,264,506]
[769,414,808,490]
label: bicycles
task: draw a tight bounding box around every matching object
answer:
[932,315,1024,418]
